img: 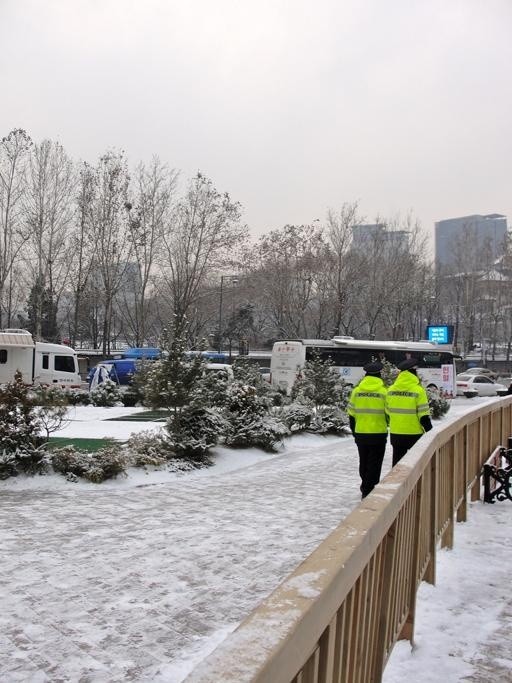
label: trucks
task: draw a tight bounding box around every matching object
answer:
[0,328,81,388]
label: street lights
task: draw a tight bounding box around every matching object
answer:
[232,278,238,335]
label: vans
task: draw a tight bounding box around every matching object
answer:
[87,359,135,385]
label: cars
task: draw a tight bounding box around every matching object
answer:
[456,374,505,396]
[203,363,234,381]
[464,368,498,380]
[260,367,271,381]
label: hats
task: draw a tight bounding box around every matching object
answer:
[397,358,418,371]
[363,362,383,373]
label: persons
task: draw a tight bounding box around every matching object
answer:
[385,358,433,468]
[346,361,388,498]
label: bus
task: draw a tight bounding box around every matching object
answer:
[271,336,461,399]
[124,347,224,362]
